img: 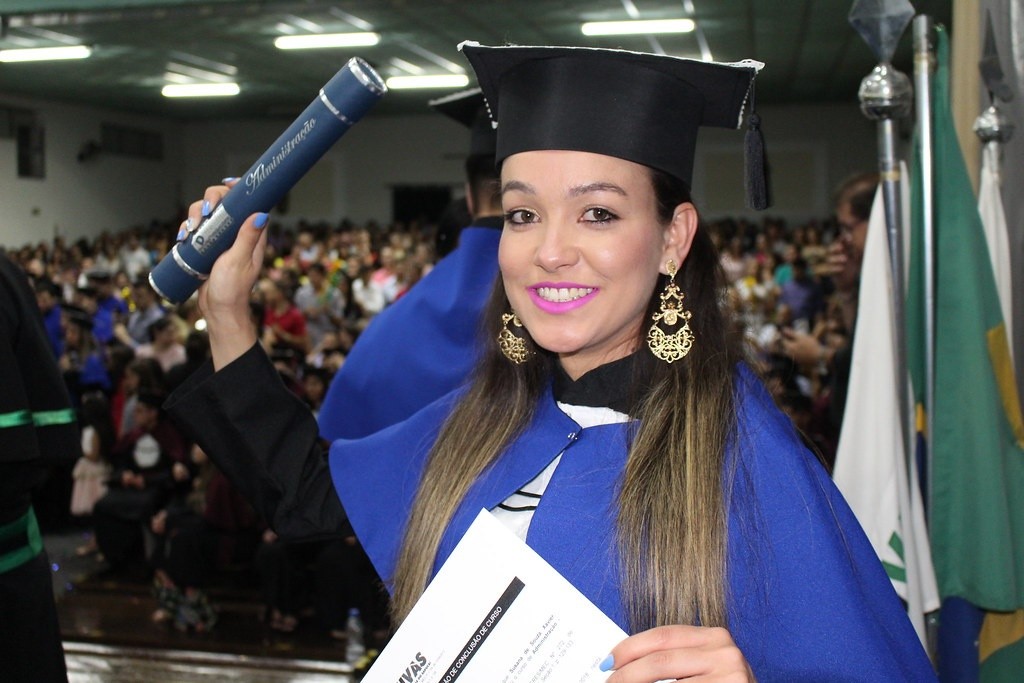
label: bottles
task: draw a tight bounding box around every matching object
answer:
[344,608,365,662]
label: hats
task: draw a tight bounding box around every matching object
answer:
[428,39,778,210]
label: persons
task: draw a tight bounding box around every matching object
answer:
[0,207,864,586]
[178,41,939,683]
[835,176,878,260]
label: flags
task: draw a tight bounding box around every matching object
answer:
[826,26,1024,660]
